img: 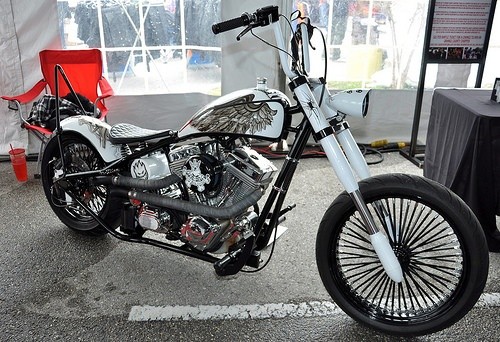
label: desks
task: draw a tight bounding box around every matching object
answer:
[423,89,500,253]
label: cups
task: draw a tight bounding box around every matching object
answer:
[9,148,28,182]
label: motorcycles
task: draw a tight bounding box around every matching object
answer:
[40,5,489,338]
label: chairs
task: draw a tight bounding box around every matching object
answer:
[0,49,114,178]
